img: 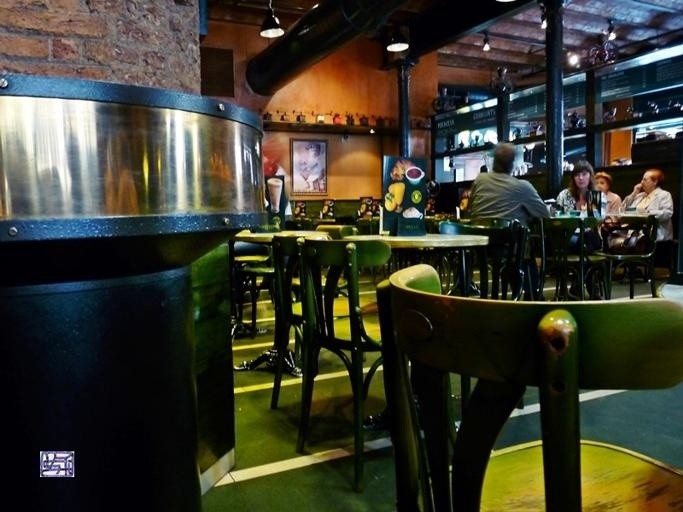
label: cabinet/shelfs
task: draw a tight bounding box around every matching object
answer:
[430,41,683,240]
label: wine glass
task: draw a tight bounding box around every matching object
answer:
[387,183,405,213]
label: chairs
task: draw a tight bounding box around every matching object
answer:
[228,200,683,512]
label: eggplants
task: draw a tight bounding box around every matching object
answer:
[384,193,397,212]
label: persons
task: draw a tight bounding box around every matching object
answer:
[298,143,324,191]
[611,168,674,284]
[466,141,552,302]
[593,171,622,251]
[554,158,608,301]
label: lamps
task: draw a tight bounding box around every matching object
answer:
[259,0,286,38]
[602,19,617,41]
[480,32,493,52]
[386,33,409,54]
[538,3,549,31]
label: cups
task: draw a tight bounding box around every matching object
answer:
[617,202,626,214]
[405,166,425,185]
[266,178,282,214]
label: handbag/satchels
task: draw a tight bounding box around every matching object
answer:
[607,227,647,255]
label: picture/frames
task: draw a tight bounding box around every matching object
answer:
[289,138,329,195]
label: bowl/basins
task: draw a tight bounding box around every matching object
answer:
[566,211,581,216]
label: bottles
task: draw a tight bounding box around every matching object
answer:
[555,204,562,216]
[550,203,556,216]
